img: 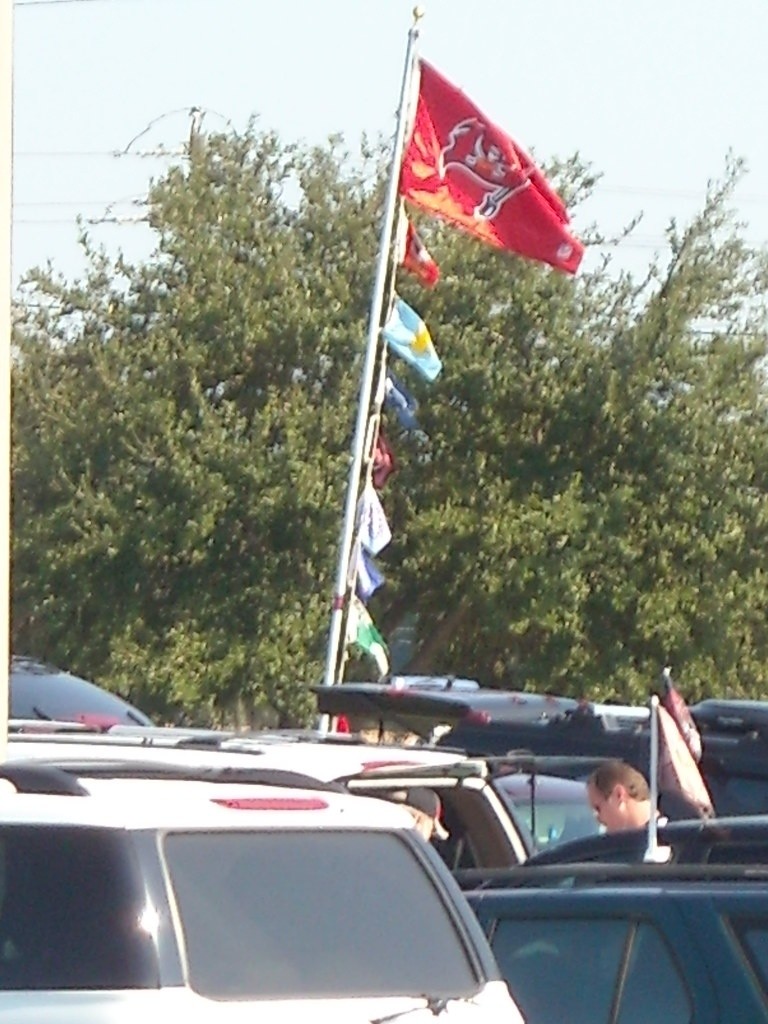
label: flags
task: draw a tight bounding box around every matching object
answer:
[398,53,584,275]
[327,209,444,733]
[654,677,713,817]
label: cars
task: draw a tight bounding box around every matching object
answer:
[1,656,767,1024]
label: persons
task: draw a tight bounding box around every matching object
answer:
[383,787,441,844]
[587,763,672,864]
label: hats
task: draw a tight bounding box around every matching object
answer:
[386,786,448,839]
